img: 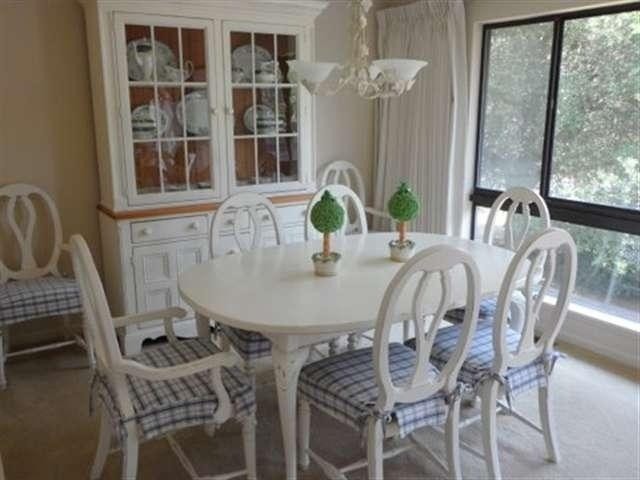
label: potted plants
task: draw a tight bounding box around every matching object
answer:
[305,188,350,279]
[380,180,421,264]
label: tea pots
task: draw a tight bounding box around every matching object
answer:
[230,57,283,82]
[131,37,154,80]
[162,58,193,82]
[133,48,157,80]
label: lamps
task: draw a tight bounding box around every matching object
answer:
[283,0,432,103]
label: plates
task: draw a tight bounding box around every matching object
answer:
[128,38,176,80]
[243,103,274,133]
[175,90,211,135]
[232,44,274,80]
[133,105,170,134]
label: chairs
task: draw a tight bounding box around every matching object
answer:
[295,242,484,478]
[1,181,93,390]
[64,231,262,480]
[209,189,341,392]
[305,185,412,354]
[440,184,552,328]
[317,160,397,238]
[401,226,579,479]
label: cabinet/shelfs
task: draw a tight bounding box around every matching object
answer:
[210,200,308,256]
[80,1,332,214]
[92,216,210,356]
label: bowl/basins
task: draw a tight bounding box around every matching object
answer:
[253,113,285,134]
[194,177,294,191]
[132,118,158,139]
